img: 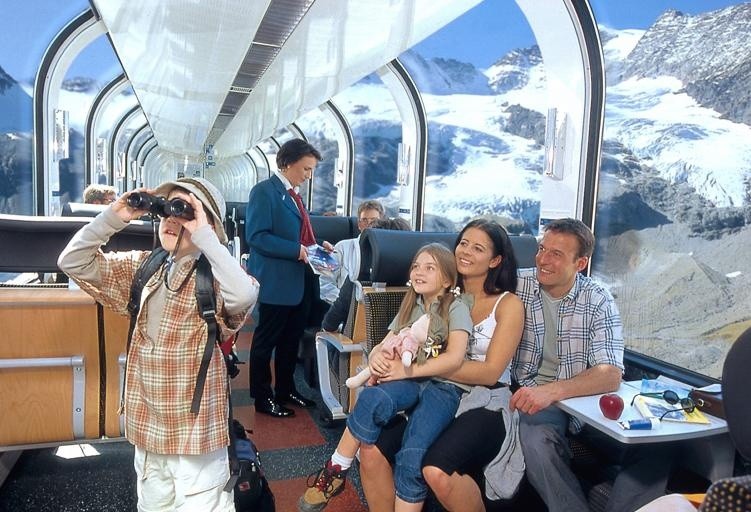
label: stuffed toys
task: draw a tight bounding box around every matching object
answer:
[345,312,448,390]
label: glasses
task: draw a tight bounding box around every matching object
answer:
[359,217,378,225]
[631,390,696,424]
[103,198,115,203]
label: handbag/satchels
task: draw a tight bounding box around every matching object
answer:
[689,383,725,419]
[223,419,277,511]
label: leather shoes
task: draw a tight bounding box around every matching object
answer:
[255,396,295,417]
[275,392,316,408]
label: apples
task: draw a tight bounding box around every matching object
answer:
[599,392,625,420]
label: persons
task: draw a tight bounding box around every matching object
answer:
[509,218,670,512]
[245,138,334,418]
[293,243,473,510]
[322,217,413,333]
[318,200,386,306]
[82,182,120,204]
[359,217,526,511]
[57,177,261,512]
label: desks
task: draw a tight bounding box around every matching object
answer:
[554,374,738,490]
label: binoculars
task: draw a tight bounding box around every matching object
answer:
[126,191,196,221]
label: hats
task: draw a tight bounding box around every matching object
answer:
[154,176,228,245]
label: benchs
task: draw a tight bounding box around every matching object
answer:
[224,201,539,452]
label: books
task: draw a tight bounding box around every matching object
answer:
[633,394,711,425]
[304,244,342,275]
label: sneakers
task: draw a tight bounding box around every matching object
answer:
[296,458,348,512]
